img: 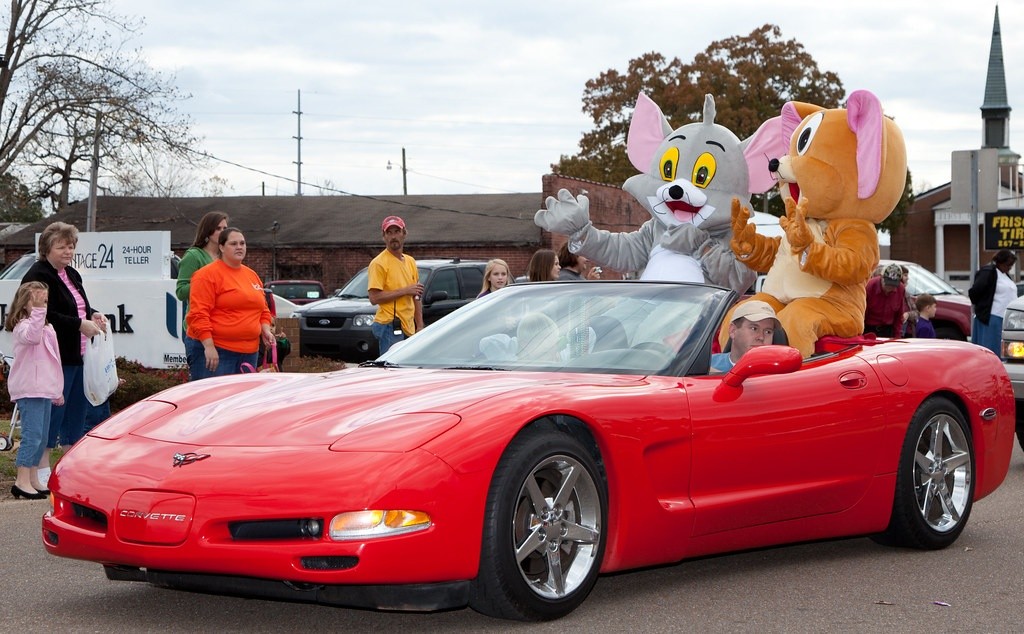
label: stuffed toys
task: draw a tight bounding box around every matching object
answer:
[535,91,784,295]
[718,90,907,358]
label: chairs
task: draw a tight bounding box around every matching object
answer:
[587,316,628,353]
[723,326,790,353]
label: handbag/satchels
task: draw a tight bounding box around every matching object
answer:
[239,343,279,373]
[82,321,118,406]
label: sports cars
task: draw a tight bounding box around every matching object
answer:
[39,276,1018,623]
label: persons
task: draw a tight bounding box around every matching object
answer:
[176,211,230,333]
[258,316,290,372]
[915,294,938,338]
[479,312,560,363]
[900,265,918,338]
[864,262,904,338]
[969,250,1019,364]
[21,222,126,488]
[709,300,782,373]
[367,215,424,357]
[559,242,600,280]
[476,258,509,299]
[527,249,561,282]
[185,227,272,376]
[5,281,65,499]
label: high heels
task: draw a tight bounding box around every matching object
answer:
[35,488,50,495]
[11,485,47,500]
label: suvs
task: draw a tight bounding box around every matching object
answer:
[290,256,517,364]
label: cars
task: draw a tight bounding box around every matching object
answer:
[262,280,330,306]
[873,259,1024,451]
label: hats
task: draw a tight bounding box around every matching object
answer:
[731,299,782,330]
[382,216,406,231]
[883,263,903,286]
